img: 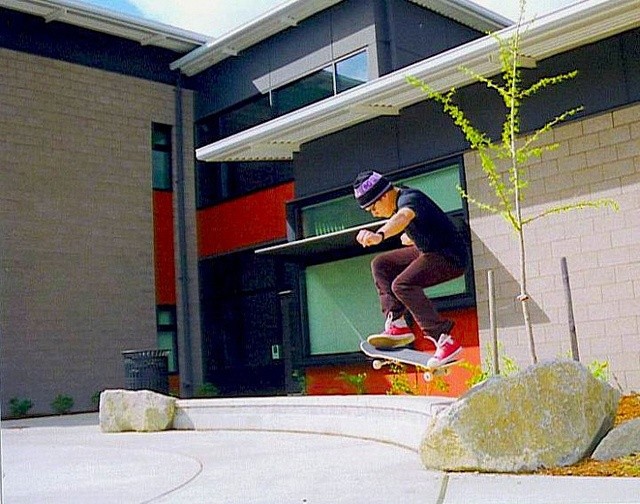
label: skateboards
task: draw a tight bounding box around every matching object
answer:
[360,339,464,381]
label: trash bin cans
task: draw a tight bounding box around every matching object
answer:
[120,349,172,395]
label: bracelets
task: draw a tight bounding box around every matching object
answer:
[375,230,386,243]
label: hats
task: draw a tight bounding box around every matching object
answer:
[354,170,391,210]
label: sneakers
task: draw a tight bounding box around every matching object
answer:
[367,312,416,350]
[423,334,463,368]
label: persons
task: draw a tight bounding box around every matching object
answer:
[352,166,465,367]
